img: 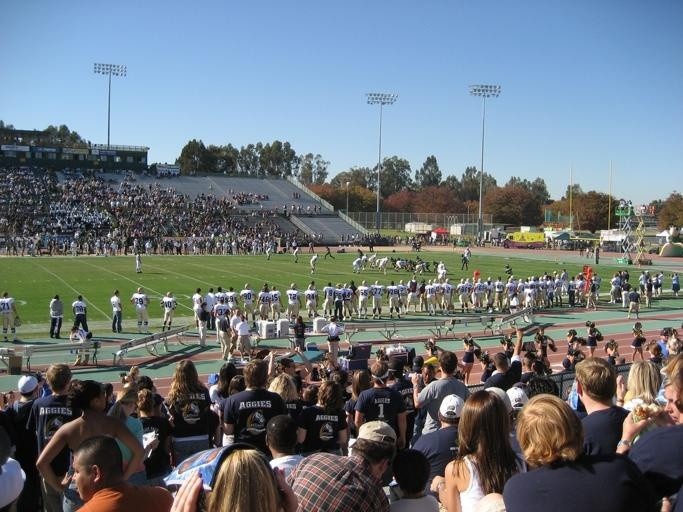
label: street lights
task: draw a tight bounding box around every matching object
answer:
[362,91,400,228]
[466,83,502,236]
[345,179,352,211]
[92,61,127,149]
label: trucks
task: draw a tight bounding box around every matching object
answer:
[501,230,546,250]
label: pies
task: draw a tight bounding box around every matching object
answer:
[632,403,662,419]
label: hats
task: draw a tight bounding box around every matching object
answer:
[439,394,463,419]
[486,387,512,415]
[0,458,26,508]
[413,355,423,372]
[506,386,528,411]
[349,420,397,446]
[17,372,41,393]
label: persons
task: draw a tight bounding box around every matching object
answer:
[0,167,682,512]
